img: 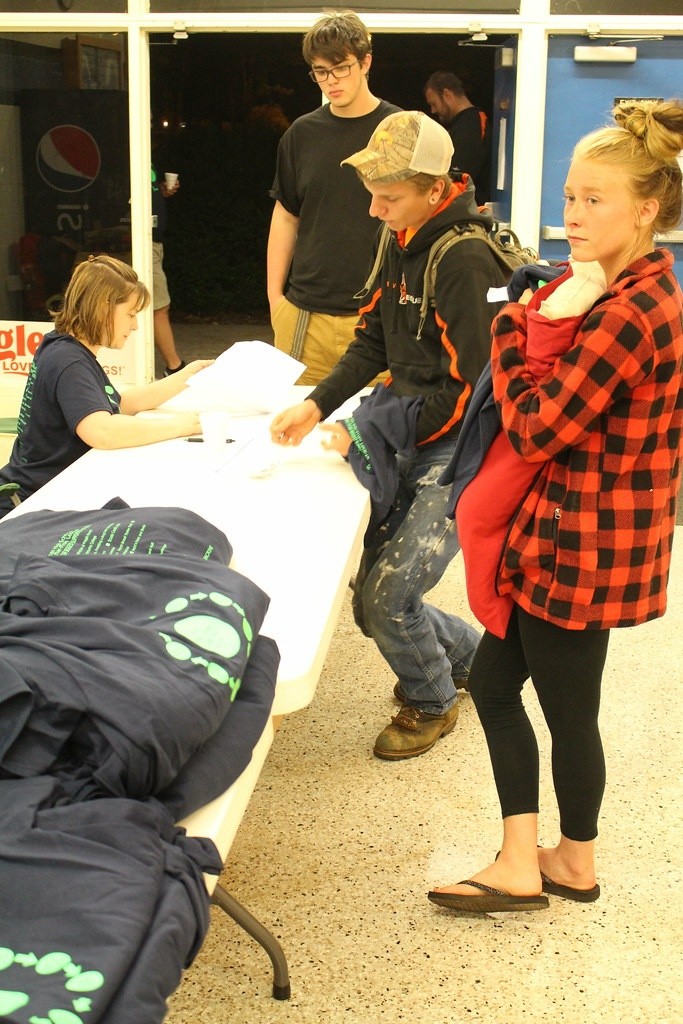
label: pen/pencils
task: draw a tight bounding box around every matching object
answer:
[182,437,236,443]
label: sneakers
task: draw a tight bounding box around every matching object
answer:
[393,679,470,703]
[373,703,458,761]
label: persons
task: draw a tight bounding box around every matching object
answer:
[0,253,215,520]
[267,9,408,387]
[151,113,196,379]
[269,110,559,760]
[427,100,683,911]
[426,70,493,206]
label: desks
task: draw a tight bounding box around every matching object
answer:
[173,715,291,1002]
[1,385,378,714]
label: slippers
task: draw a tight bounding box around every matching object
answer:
[495,845,601,902]
[428,880,549,912]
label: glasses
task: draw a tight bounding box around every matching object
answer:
[308,58,364,83]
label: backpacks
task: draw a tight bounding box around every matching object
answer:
[372,224,540,339]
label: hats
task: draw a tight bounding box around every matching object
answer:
[340,111,454,184]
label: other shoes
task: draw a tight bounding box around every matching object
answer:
[164,360,186,377]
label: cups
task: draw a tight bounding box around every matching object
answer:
[165,173,179,190]
